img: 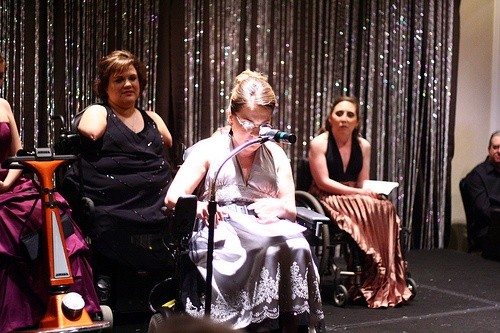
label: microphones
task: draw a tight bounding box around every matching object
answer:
[258,127,296,144]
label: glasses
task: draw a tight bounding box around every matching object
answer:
[234,112,272,132]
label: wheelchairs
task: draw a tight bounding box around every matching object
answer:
[0,114,188,333]
[140,191,331,333]
[294,156,418,308]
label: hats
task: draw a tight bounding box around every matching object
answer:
[202,220,247,276]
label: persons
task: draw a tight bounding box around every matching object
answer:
[308,96,412,309]
[163,70,324,333]
[464,131,500,264]
[62,50,205,333]
[0,52,101,333]
